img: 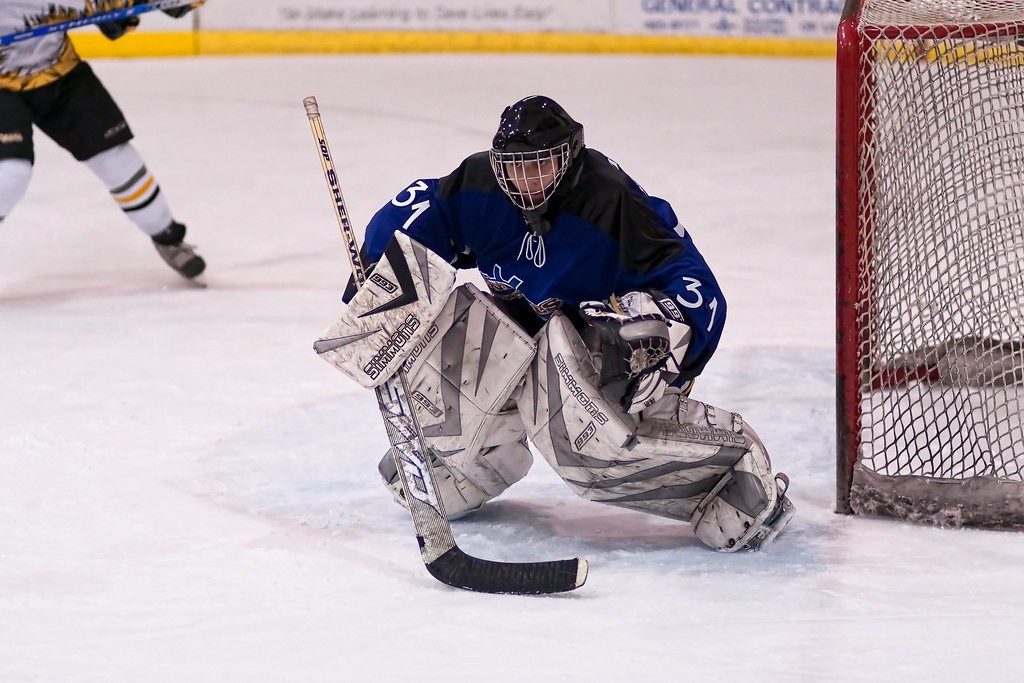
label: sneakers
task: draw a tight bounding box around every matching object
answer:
[151,220,207,289]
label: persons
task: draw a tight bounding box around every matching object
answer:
[0,0,205,280]
[342,98,794,556]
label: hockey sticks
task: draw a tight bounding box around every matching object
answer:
[3,0,198,47]
[298,93,590,598]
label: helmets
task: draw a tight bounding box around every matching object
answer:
[489,95,586,236]
